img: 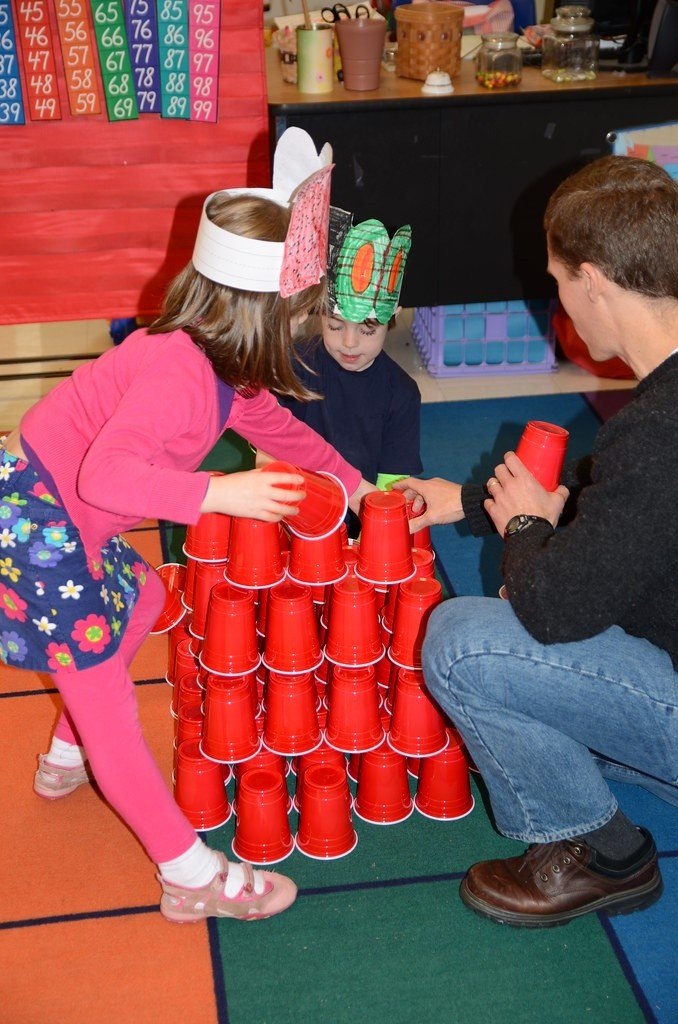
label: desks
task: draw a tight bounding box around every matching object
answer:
[266,60,678,305]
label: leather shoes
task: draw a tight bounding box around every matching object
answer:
[459,825,664,929]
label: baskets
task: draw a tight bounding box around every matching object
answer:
[395,1,464,83]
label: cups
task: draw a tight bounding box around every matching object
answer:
[508,420,570,495]
[146,460,476,863]
[334,20,387,91]
[296,24,332,94]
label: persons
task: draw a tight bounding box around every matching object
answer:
[392,154,677,931]
[0,125,382,922]
[248,206,424,542]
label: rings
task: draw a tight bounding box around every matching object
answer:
[488,482,500,495]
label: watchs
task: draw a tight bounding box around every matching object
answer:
[504,514,554,538]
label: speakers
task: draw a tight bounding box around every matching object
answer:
[646,0,678,79]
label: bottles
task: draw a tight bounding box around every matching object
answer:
[541,5,598,82]
[475,32,523,89]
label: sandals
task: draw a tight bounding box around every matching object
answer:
[155,849,297,924]
[33,754,95,801]
